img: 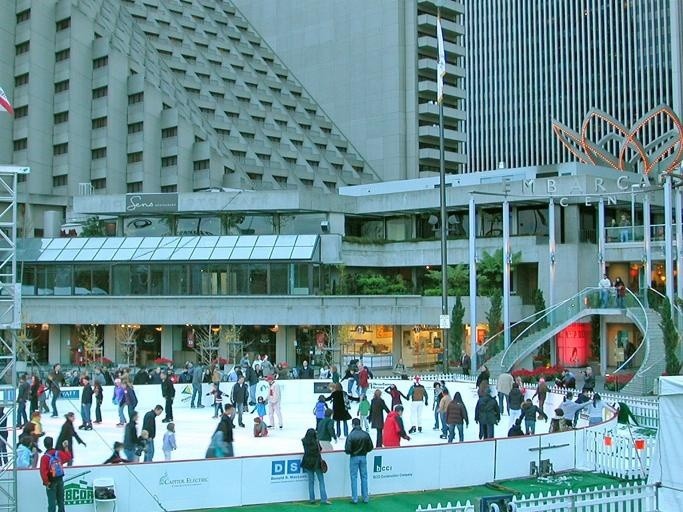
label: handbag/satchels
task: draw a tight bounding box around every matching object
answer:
[321,460,327,473]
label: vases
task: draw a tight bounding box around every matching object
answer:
[609,383,624,391]
[537,374,554,381]
[514,374,533,383]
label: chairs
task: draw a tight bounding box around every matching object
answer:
[92,477,119,512]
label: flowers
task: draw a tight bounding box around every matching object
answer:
[554,364,568,373]
[512,368,534,376]
[534,367,555,376]
[607,373,635,384]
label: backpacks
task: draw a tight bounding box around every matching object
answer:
[44,450,64,479]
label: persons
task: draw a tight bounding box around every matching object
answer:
[300,428,332,504]
[345,418,373,504]
[103,353,283,463]
[474,365,617,435]
[620,215,630,243]
[614,276,626,308]
[599,273,612,308]
[610,218,620,242]
[0,339,103,512]
[569,348,578,368]
[299,359,469,450]
[621,338,637,368]
